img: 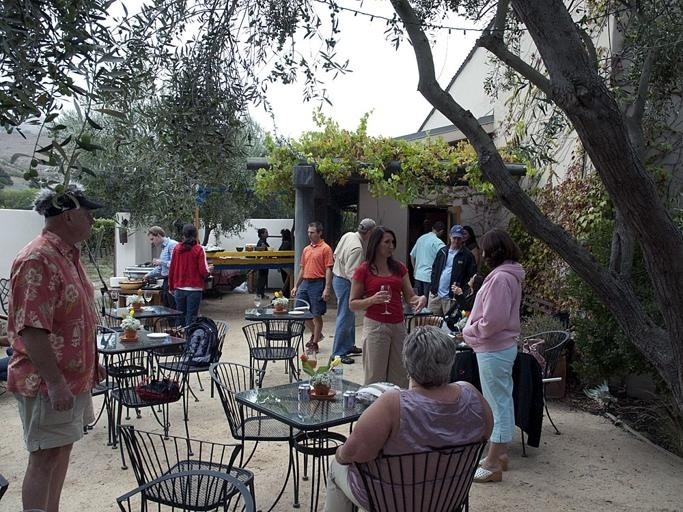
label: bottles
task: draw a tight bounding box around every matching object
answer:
[331,356,343,392]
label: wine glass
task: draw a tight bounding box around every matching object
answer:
[451,280,460,302]
[253,293,260,318]
[143,290,152,311]
[380,284,391,315]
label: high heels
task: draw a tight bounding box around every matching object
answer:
[474,462,502,483]
[478,454,508,471]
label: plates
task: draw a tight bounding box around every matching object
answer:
[310,390,337,400]
[272,309,287,314]
[119,335,139,341]
[128,308,143,311]
[287,310,305,316]
[145,332,170,339]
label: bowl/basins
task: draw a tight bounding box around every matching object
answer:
[255,247,265,251]
[267,247,274,252]
[119,281,145,290]
[235,247,243,252]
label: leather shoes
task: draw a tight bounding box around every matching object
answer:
[347,346,362,354]
[332,355,354,364]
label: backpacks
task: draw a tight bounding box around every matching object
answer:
[180,316,222,366]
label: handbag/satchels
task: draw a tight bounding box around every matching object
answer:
[153,326,187,354]
[354,382,403,407]
[135,375,180,401]
[522,339,551,378]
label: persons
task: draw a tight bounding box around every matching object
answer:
[3,178,102,510]
[290,220,334,353]
[167,223,216,331]
[142,225,180,324]
[276,228,292,288]
[255,227,271,301]
[322,323,494,511]
[461,228,526,484]
[327,217,494,391]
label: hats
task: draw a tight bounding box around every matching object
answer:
[450,225,464,239]
[358,218,376,233]
[43,190,105,218]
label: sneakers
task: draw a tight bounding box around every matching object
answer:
[306,342,319,353]
[306,333,324,348]
